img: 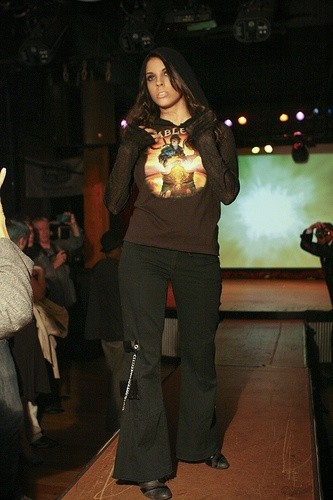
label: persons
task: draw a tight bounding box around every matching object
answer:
[5,211,82,447]
[101,48,241,500]
[299,222,333,307]
[83,230,122,435]
[0,167,32,500]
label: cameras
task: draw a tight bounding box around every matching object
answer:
[317,228,330,239]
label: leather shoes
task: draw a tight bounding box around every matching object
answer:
[137,479,172,500]
[205,452,229,469]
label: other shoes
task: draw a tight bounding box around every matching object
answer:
[32,432,59,451]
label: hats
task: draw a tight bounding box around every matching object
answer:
[99,230,123,252]
